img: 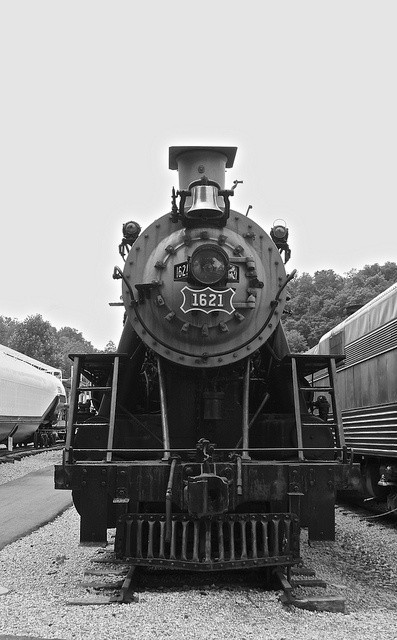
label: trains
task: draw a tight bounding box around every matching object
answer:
[52,144,350,581]
[0,345,66,449]
[299,284,396,523]
